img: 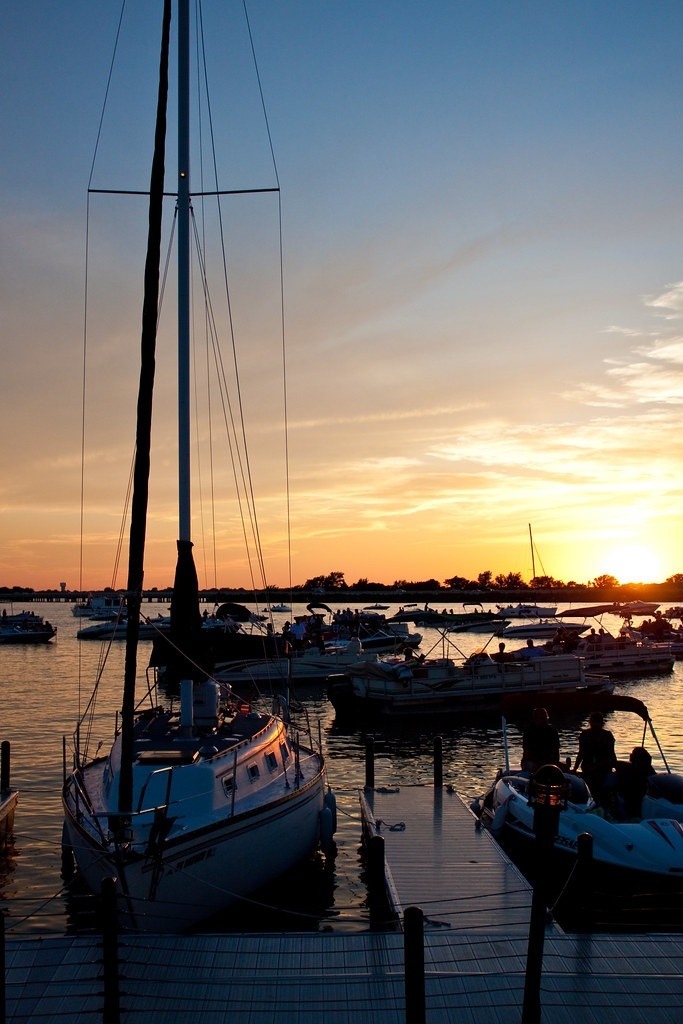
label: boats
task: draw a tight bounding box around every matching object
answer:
[0,739,21,857]
[72,519,683,716]
[0,607,57,644]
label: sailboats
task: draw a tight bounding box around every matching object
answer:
[63,2,334,933]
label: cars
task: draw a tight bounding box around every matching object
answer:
[476,684,682,901]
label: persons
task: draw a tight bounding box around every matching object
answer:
[517,706,657,822]
[117,606,683,670]
